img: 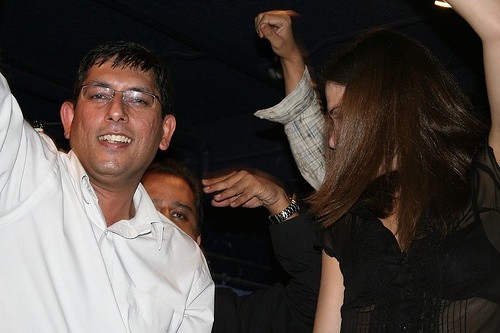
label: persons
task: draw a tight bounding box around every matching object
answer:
[0,41,216,333]
[253,0,500,333]
[140,161,317,333]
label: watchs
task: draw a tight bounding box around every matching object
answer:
[269,193,300,222]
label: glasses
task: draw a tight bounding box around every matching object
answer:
[79,84,167,111]
